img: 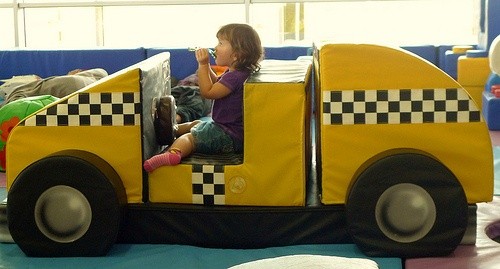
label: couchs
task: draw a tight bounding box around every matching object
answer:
[146,54,315,208]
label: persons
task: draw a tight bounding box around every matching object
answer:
[0,67,109,108]
[171,68,217,123]
[143,23,264,172]
